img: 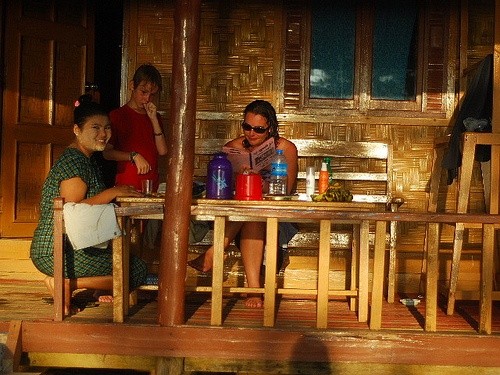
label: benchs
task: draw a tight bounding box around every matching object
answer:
[147,138,404,303]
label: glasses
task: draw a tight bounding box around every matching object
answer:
[241,118,271,134]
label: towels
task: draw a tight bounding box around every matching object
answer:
[62,201,122,251]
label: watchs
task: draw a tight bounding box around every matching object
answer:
[129,151,139,163]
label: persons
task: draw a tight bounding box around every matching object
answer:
[189,100,298,308]
[29,94,148,312]
[103,65,168,194]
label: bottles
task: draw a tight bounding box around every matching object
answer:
[269,149,288,195]
[206,152,233,200]
[306,166,315,196]
[324,158,332,186]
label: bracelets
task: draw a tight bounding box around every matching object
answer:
[153,128,165,135]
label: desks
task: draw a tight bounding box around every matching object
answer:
[115,195,374,329]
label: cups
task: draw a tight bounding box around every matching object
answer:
[141,179,153,194]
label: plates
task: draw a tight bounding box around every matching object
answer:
[399,299,420,306]
